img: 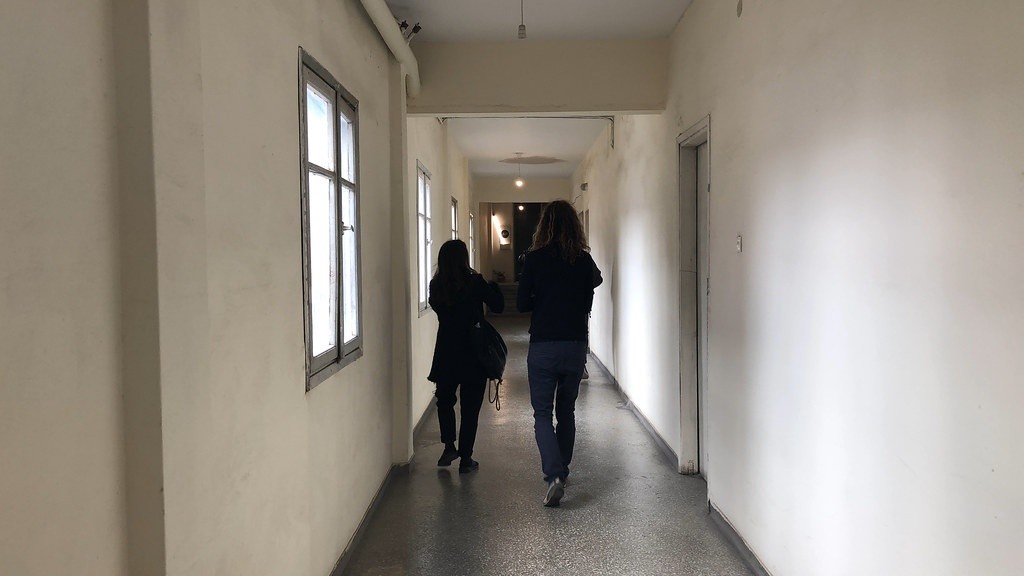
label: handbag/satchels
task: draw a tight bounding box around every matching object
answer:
[471,320,507,383]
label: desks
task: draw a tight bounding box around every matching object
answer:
[487,281,532,321]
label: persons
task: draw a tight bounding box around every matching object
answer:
[427,239,504,474]
[514,199,604,507]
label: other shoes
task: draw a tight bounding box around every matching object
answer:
[459,460,479,475]
[438,447,458,466]
[543,477,567,507]
